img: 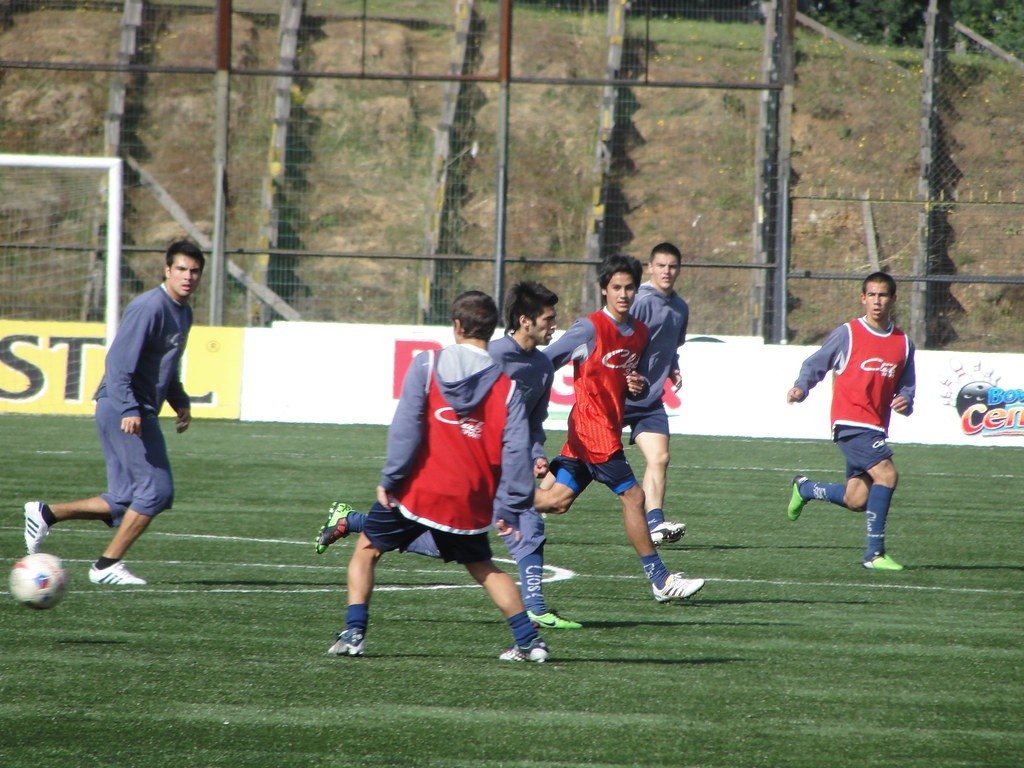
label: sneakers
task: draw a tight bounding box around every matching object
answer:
[652,571,705,604]
[527,609,583,630]
[862,553,904,572]
[315,501,355,554]
[499,636,550,664]
[787,475,810,522]
[649,520,687,547]
[88,561,147,586]
[327,628,366,657]
[23,501,60,556]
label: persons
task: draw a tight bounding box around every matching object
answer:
[23,241,206,585]
[787,274,916,572]
[537,242,703,603]
[315,281,581,664]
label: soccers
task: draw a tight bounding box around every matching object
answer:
[9,553,67,609]
